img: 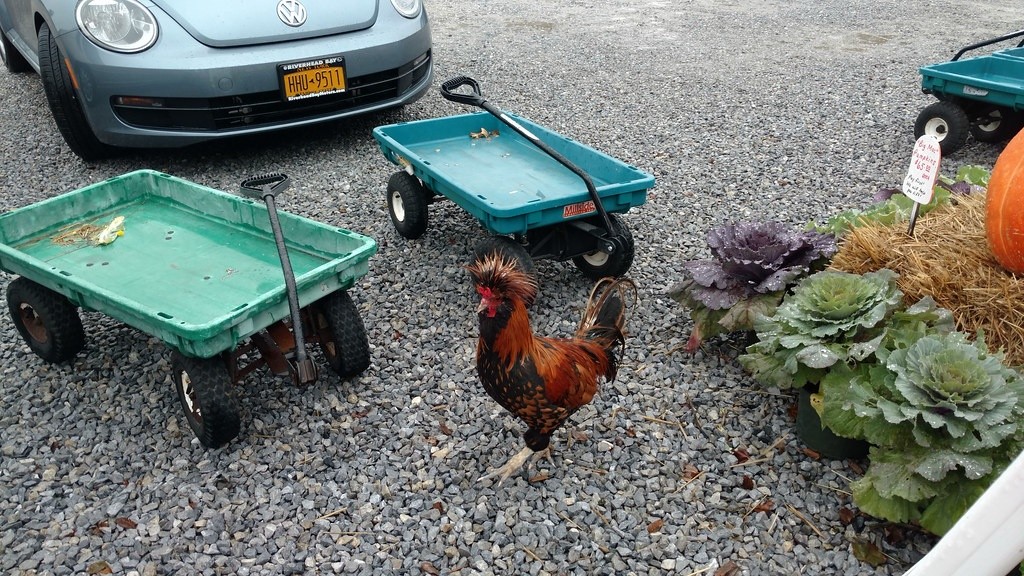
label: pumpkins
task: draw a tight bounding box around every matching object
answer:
[984,125,1024,276]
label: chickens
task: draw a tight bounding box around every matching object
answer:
[461,246,638,489]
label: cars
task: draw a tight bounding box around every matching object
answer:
[0,0,435,162]
